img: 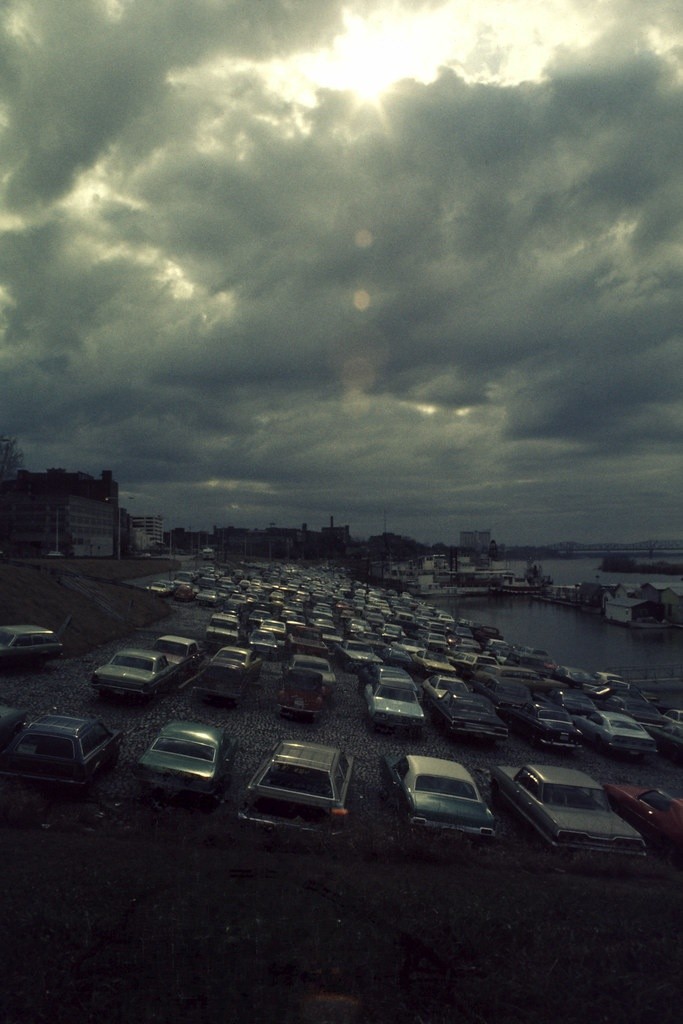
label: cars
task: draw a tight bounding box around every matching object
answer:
[605,780,683,857]
[245,736,355,815]
[0,711,125,788]
[46,550,65,560]
[385,750,496,843]
[0,624,65,671]
[133,719,238,807]
[494,760,648,861]
[88,546,683,766]
